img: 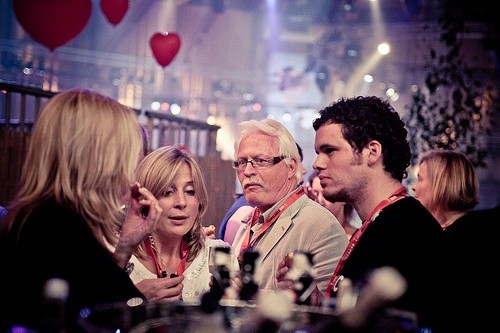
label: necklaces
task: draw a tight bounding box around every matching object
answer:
[442,213,463,227]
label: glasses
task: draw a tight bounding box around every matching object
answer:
[232,156,286,169]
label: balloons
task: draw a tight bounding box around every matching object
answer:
[150,31,181,68]
[100,0,129,26]
[12,0,93,52]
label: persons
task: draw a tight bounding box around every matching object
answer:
[312,96,446,333]
[203,118,349,309]
[412,148,500,333]
[218,142,324,246]
[308,170,359,241]
[0,88,164,333]
[100,145,240,308]
